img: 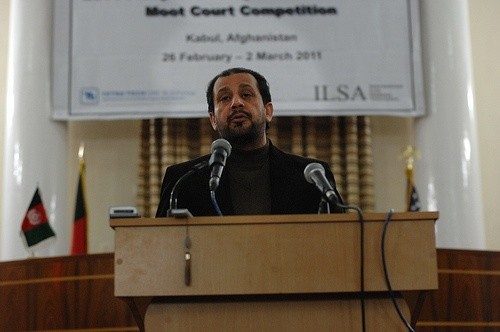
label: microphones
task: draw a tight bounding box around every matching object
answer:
[304,162,338,204]
[209,138,232,216]
[167,160,209,217]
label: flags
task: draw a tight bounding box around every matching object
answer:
[70,164,88,256]
[19,183,58,254]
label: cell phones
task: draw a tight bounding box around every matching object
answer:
[171,208,194,218]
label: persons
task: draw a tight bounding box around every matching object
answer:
[154,68,347,218]
